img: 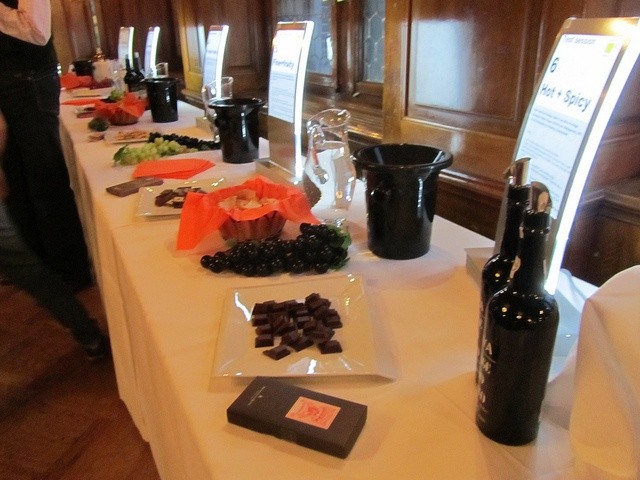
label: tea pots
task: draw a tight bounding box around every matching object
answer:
[146,62,169,79]
[202,76,234,140]
[303,108,356,228]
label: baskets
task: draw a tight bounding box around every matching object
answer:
[108,108,138,125]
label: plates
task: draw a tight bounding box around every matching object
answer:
[105,129,151,143]
[64,85,114,119]
[133,177,227,217]
[210,273,397,380]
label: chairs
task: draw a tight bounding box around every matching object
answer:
[569,266,639,480]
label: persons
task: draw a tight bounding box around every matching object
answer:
[0,0,90,289]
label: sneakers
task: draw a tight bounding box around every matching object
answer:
[81,318,105,362]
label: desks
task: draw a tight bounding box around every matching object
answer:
[57,80,602,480]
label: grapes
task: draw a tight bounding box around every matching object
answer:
[112,134,221,165]
[200,224,352,277]
[111,89,124,100]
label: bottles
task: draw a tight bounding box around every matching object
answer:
[475,182,559,445]
[128,52,147,100]
[474,157,532,385]
[124,54,133,93]
